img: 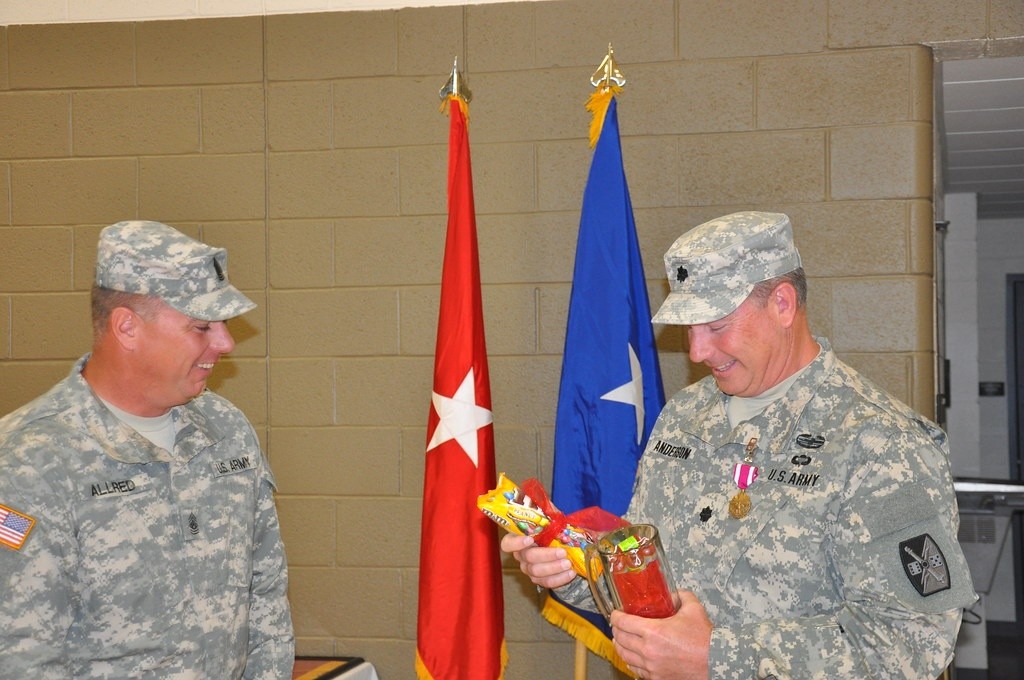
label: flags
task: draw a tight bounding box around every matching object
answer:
[551,42,667,677]
[412,58,508,680]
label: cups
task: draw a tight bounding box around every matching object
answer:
[583,524,682,619]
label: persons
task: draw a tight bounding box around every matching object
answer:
[0,220,295,680]
[500,210,978,680]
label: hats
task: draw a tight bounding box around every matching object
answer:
[92,219,258,321]
[651,212,803,325]
